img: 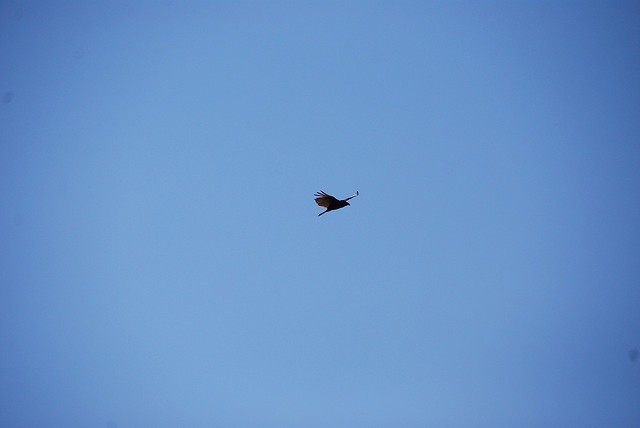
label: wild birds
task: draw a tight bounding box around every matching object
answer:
[314,187,360,217]
